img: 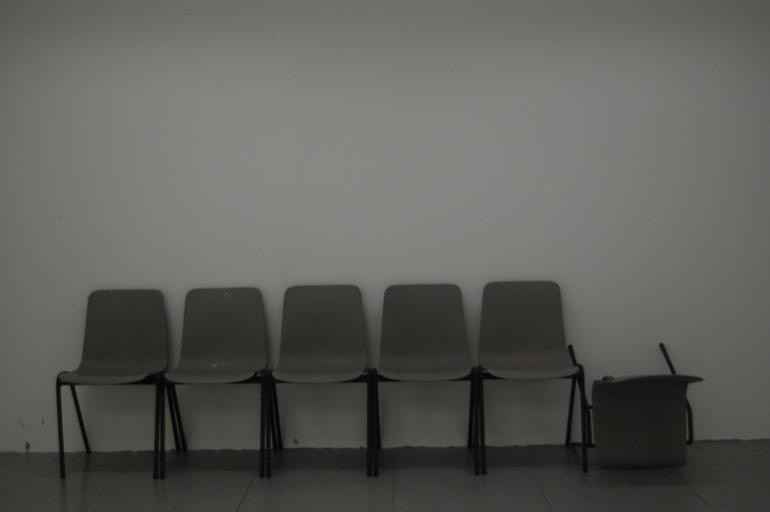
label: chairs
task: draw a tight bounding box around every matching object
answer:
[265,283,374,479]
[478,279,590,476]
[56,287,182,481]
[374,282,483,478]
[159,285,279,483]
[564,343,706,486]
